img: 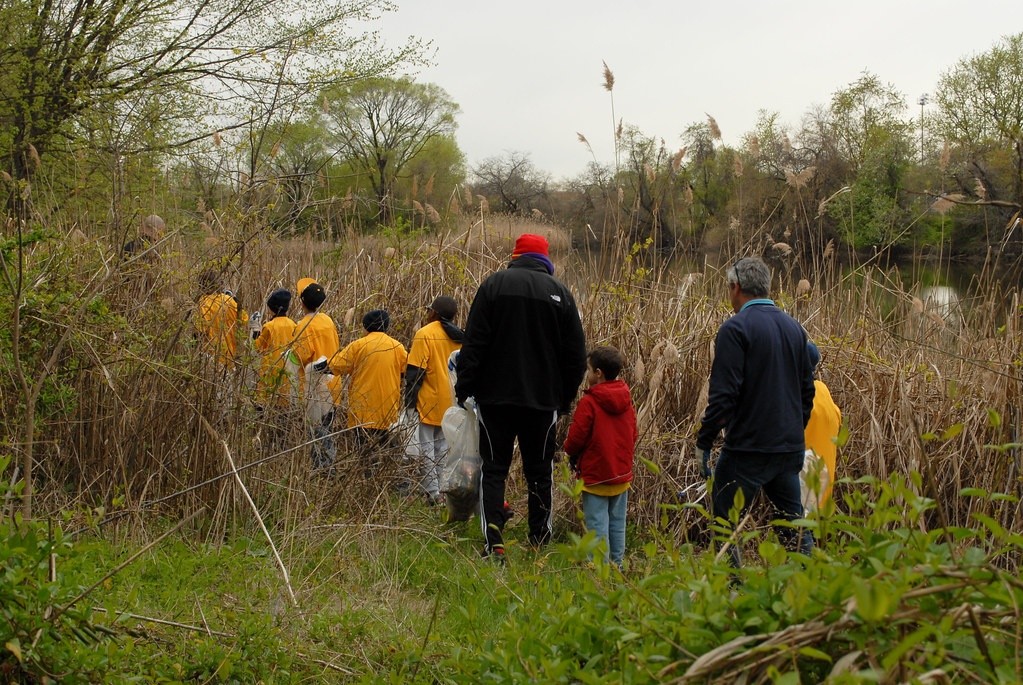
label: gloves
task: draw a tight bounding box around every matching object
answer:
[695,447,712,479]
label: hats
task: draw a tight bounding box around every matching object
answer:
[363,310,389,332]
[512,232,548,258]
[296,277,326,305]
[267,288,291,313]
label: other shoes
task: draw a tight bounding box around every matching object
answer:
[494,551,505,565]
[504,505,514,523]
[424,494,444,507]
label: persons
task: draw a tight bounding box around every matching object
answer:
[453,232,587,568]
[313,309,410,500]
[404,296,466,507]
[563,346,640,576]
[288,278,343,482]
[190,269,250,371]
[695,256,818,597]
[250,287,298,453]
[797,341,842,551]
[119,215,166,302]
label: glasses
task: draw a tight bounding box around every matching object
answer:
[426,305,438,313]
[733,258,744,286]
